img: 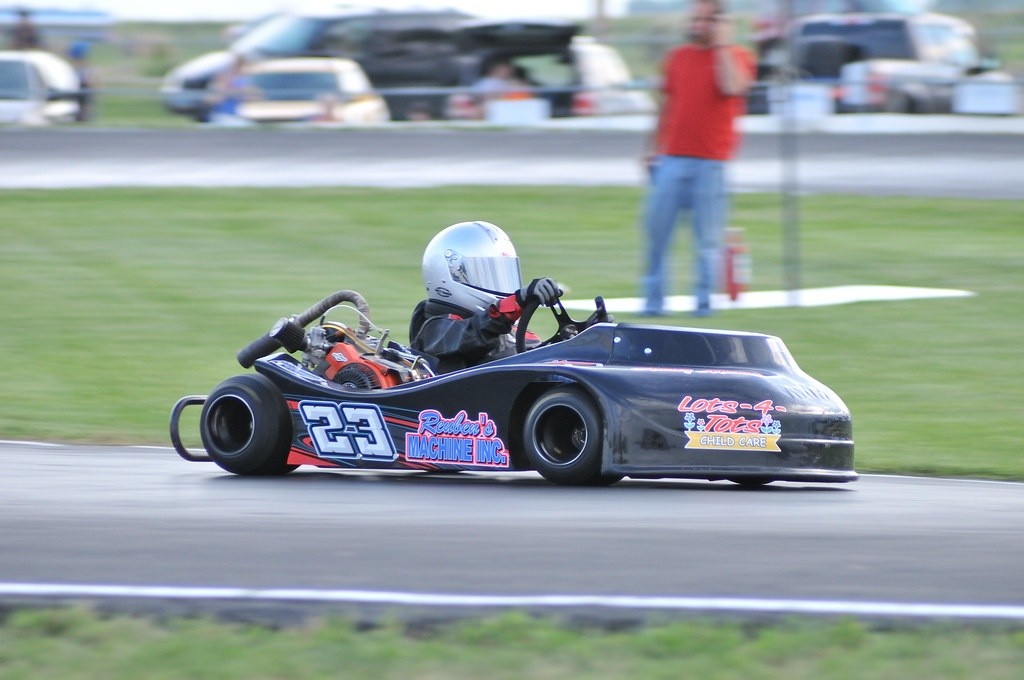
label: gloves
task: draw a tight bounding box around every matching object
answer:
[515,277,563,307]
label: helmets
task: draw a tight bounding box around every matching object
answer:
[422,221,523,325]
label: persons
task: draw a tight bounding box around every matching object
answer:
[634,0,757,319]
[409,220,615,379]
[404,101,432,122]
[305,93,343,123]
[469,60,532,98]
[68,38,94,122]
[202,54,260,126]
[13,11,44,51]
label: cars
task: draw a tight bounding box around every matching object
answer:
[154,0,1023,124]
[200,52,394,122]
[0,48,86,126]
